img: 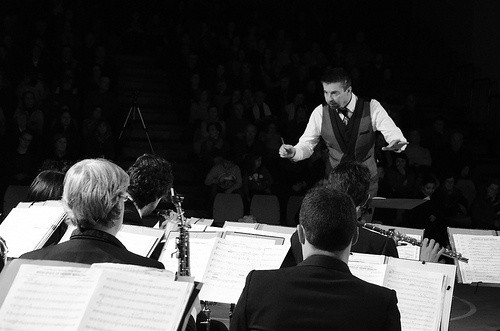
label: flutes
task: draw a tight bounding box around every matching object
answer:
[357,220,470,264]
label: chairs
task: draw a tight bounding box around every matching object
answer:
[250,195,281,225]
[286,196,304,226]
[213,194,243,227]
[3,184,28,218]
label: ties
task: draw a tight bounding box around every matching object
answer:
[338,108,349,127]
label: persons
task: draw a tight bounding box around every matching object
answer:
[277,69,410,225]
[0,0,500,231]
[288,163,446,264]
[229,189,401,331]
[0,155,227,331]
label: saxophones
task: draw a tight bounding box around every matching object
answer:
[168,186,213,331]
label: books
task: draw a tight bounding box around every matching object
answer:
[0,201,500,331]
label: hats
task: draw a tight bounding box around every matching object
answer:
[216,173,236,189]
